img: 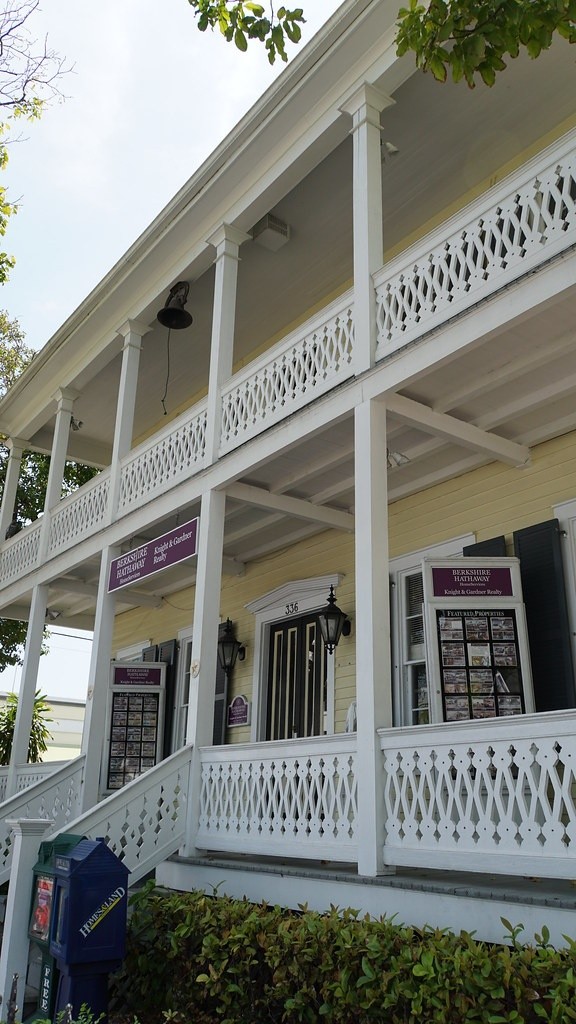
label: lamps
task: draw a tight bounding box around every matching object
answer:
[217,617,245,677]
[46,608,62,622]
[70,416,84,432]
[317,583,351,655]
[253,215,291,251]
[380,137,400,163]
[386,447,412,468]
